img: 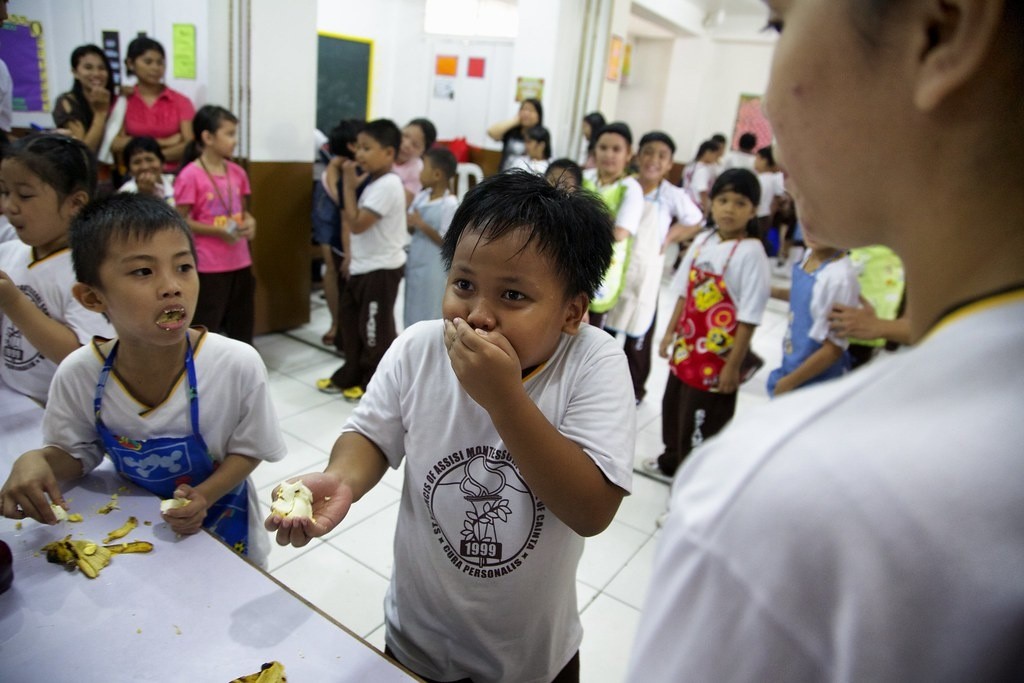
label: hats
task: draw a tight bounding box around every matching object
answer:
[592,122,632,145]
[709,167,761,208]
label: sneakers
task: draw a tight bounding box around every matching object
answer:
[318,378,344,394]
[323,334,336,347]
[341,384,365,401]
[631,454,675,487]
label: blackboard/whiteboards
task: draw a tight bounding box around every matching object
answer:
[316,29,375,138]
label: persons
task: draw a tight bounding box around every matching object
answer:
[264,168,637,683]
[0,37,257,405]
[314,100,915,479]
[620,0,1023,683]
[0,192,288,570]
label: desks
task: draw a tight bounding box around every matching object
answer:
[0,382,422,683]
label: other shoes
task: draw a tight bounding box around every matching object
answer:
[769,264,791,280]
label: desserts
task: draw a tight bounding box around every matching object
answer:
[161,499,190,511]
[272,484,314,523]
[52,506,68,520]
